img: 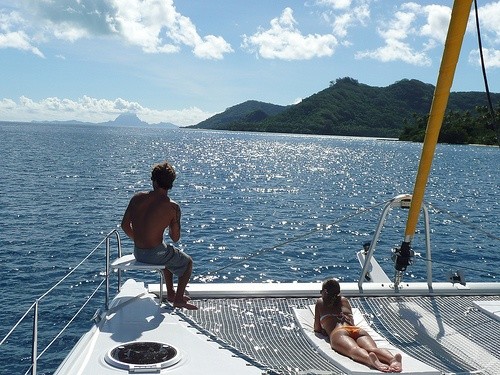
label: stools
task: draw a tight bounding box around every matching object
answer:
[111,253,166,306]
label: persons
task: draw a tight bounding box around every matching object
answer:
[313,280,402,372]
[121,161,199,311]
